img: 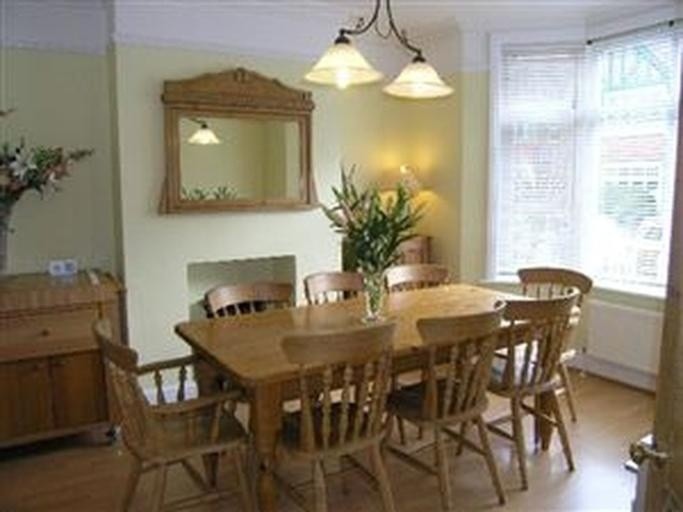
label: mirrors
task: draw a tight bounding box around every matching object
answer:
[158,67,324,215]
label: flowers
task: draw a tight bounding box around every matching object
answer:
[0,108,94,239]
[322,162,429,312]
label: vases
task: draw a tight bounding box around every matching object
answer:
[357,268,389,325]
[0,195,17,278]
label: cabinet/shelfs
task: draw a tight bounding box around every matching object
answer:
[0,307,119,450]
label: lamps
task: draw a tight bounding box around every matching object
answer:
[304,0,457,100]
[188,118,222,144]
